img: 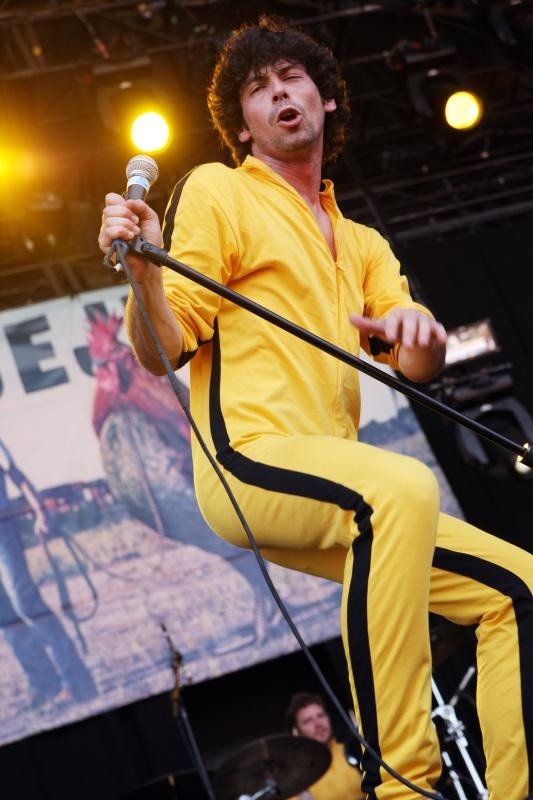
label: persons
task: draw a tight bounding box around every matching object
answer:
[97,24,533,800]
[0,440,98,706]
[286,692,367,800]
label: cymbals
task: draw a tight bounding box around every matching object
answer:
[204,734,333,800]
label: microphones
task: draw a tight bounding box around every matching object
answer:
[112,155,159,271]
